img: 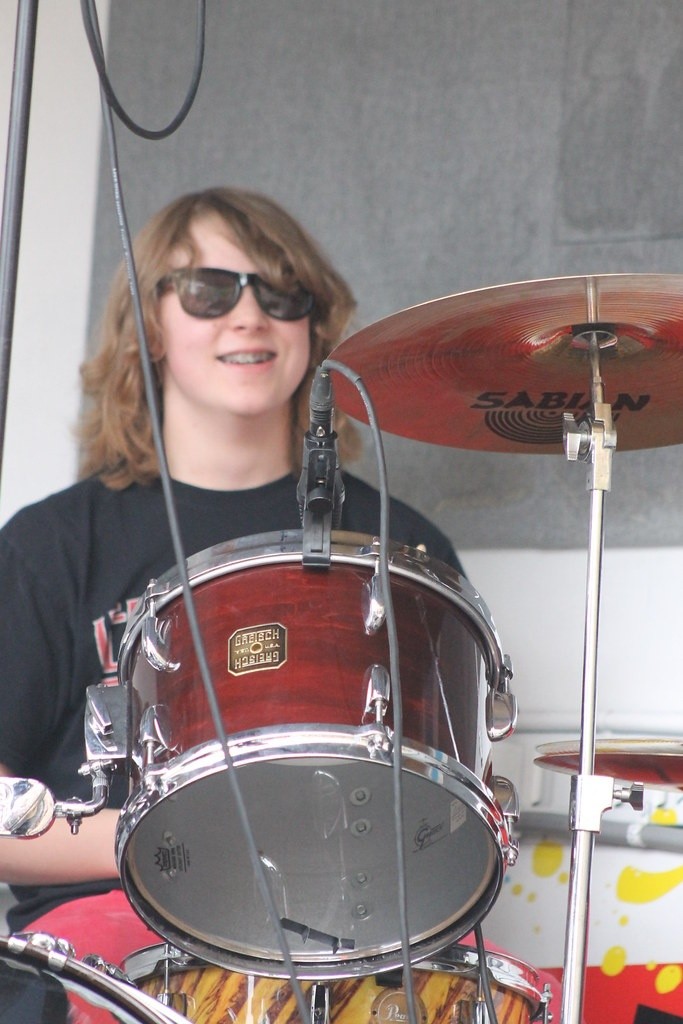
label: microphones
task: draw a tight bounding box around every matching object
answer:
[296,363,345,529]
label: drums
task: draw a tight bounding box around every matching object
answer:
[121,942,553,1024]
[113,526,520,982]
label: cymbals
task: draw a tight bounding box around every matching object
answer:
[324,273,683,453]
[533,739,683,786]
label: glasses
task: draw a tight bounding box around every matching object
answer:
[155,266,316,321]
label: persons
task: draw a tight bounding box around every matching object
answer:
[0,187,468,1024]
